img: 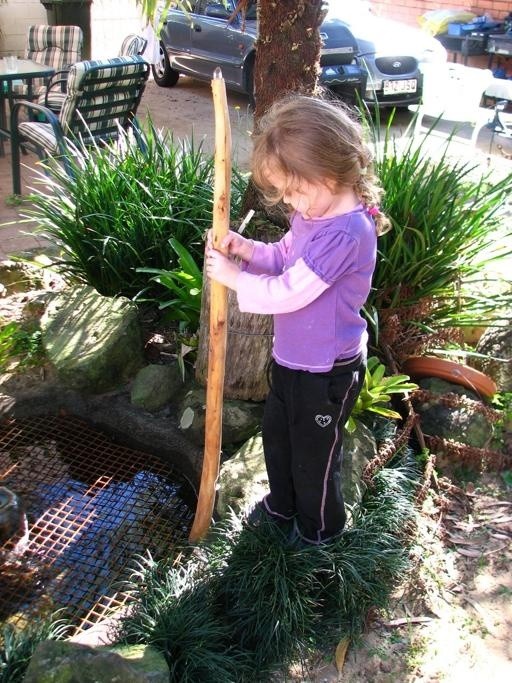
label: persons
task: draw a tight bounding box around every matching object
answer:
[203,96,394,545]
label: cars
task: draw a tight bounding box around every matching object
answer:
[151,0,423,112]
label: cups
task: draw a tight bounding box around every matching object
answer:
[5,55,19,73]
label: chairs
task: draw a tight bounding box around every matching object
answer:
[0,24,150,207]
